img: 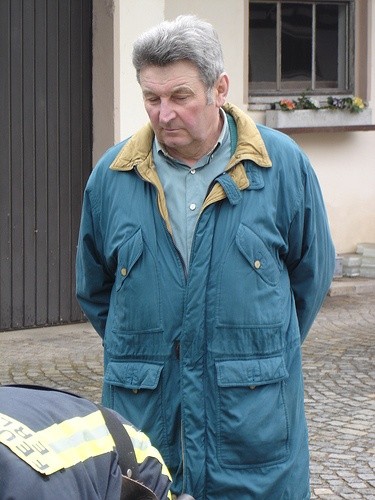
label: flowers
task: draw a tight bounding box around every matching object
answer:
[268,91,366,115]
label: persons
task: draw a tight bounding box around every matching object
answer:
[0,383,173,500]
[75,16,336,500]
